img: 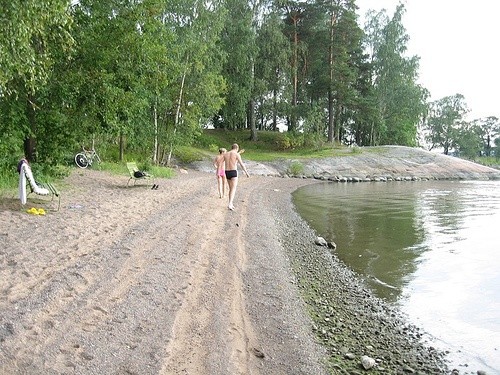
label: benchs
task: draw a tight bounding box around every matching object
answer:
[18,158,60,212]
[126,161,155,190]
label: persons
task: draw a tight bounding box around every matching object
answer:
[213,148,245,198]
[218,143,249,211]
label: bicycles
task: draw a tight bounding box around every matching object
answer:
[74,144,101,169]
